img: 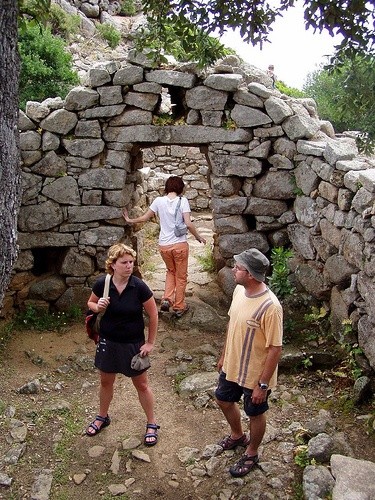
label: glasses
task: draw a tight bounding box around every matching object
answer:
[234,262,246,272]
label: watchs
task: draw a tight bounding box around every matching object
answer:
[258,381,268,391]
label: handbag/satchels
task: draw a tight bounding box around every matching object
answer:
[84,313,100,346]
[174,223,188,236]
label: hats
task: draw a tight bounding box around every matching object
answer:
[233,248,269,283]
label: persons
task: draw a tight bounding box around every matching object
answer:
[215,247,283,477]
[122,176,206,317]
[86,244,160,446]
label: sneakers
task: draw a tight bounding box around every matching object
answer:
[160,300,169,312]
[175,304,189,317]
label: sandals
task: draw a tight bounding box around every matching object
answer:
[219,433,249,450]
[230,451,259,476]
[86,414,110,436]
[144,421,161,446]
